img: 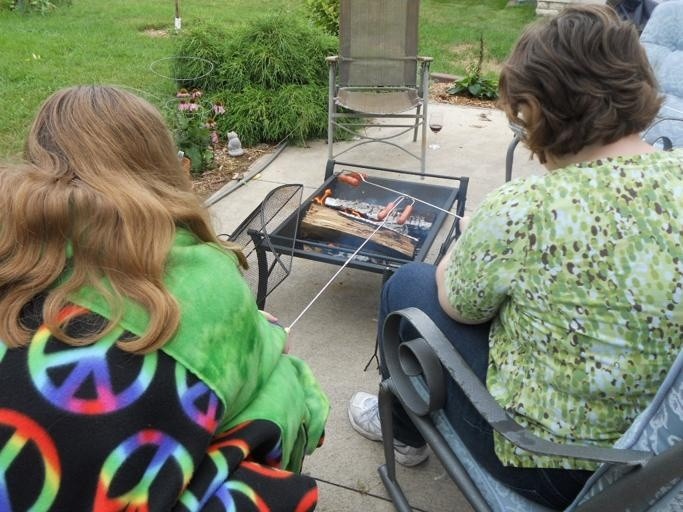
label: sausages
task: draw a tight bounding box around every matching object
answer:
[398,204,413,225]
[338,171,366,187]
[377,202,394,220]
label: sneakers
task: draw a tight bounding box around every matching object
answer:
[347,390,433,468]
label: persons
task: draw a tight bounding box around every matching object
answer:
[0,86,331,512]
[348,3,683,512]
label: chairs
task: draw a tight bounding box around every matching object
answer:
[373,299,681,512]
[326,1,434,178]
